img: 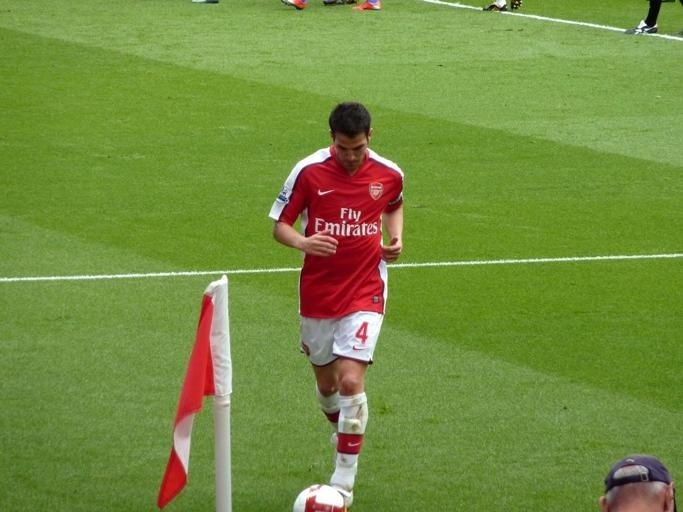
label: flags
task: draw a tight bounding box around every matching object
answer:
[157,275,233,511]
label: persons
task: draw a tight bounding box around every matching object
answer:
[279,0,522,11]
[268,102,405,509]
[625,0,683,34]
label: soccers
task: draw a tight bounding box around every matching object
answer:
[293,484,346,512]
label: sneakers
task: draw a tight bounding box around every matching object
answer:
[330,481,354,510]
[352,0,382,11]
[483,1,508,11]
[624,19,658,35]
[280,0,305,10]
[511,0,522,11]
